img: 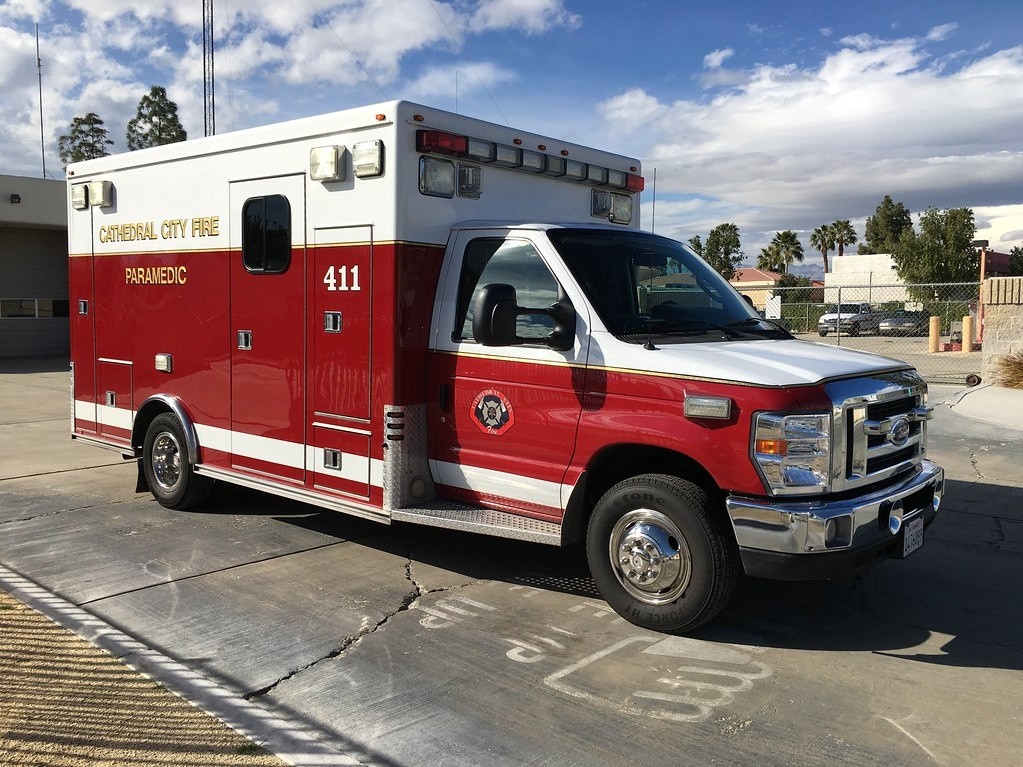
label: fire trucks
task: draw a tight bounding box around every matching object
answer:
[66,100,947,635]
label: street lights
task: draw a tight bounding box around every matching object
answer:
[971,239,990,341]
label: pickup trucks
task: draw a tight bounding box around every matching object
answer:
[818,300,892,338]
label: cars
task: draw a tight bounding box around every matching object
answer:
[879,309,933,338]
